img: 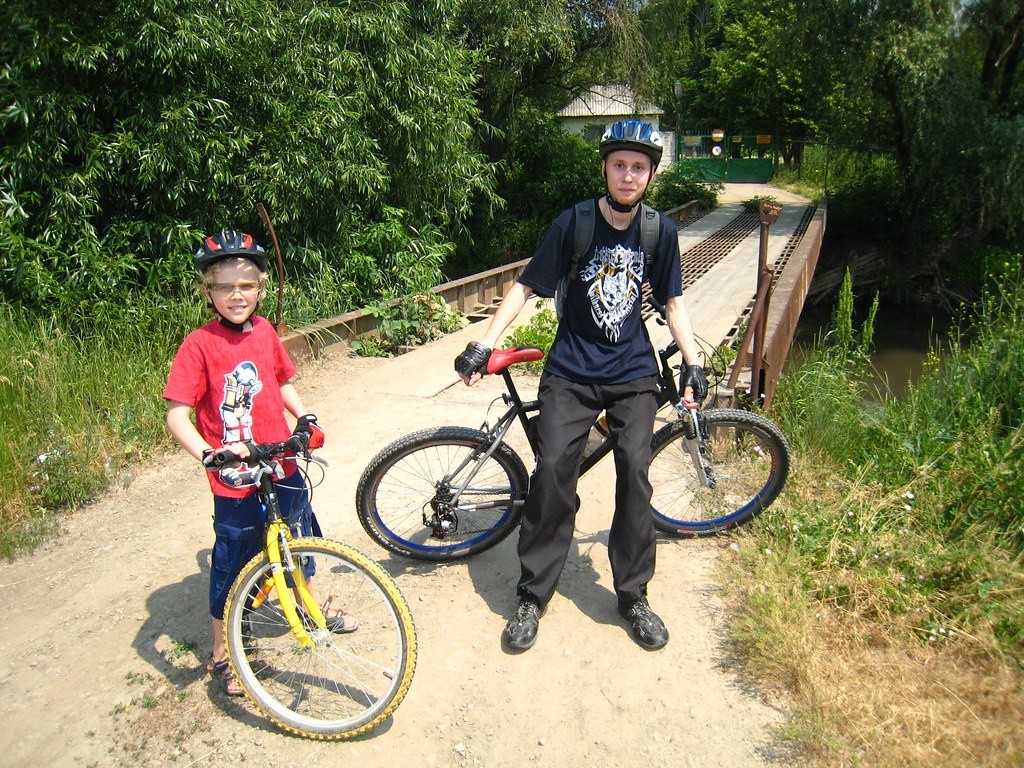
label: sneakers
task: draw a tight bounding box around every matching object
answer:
[618,594,669,651]
[503,587,548,650]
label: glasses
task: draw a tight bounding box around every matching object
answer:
[207,283,264,297]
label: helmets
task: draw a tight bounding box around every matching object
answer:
[599,119,663,164]
[192,230,266,272]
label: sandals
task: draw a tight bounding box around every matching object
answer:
[295,595,358,633]
[206,651,246,696]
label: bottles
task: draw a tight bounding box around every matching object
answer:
[578,416,611,465]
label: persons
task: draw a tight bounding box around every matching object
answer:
[454,119,708,651]
[162,230,358,696]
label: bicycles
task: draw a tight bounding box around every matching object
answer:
[355,297,793,562]
[215,430,418,742]
